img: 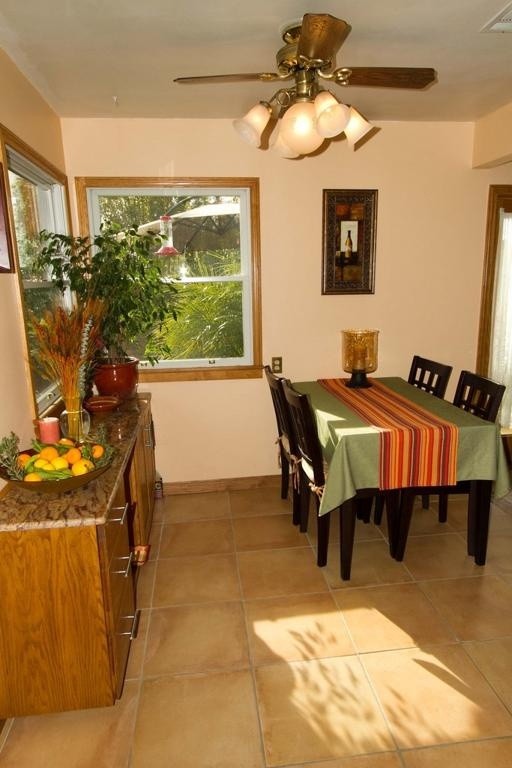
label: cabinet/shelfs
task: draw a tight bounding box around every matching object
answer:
[90,386,161,580]
[1,403,139,722]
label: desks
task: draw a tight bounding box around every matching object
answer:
[285,372,511,584]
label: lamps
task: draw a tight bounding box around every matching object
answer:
[225,71,381,163]
[341,326,382,390]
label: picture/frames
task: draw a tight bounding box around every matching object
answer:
[320,185,379,298]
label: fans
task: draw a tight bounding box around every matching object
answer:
[162,11,437,97]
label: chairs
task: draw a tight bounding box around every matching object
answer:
[373,351,453,528]
[395,368,506,565]
[275,375,401,565]
[262,359,371,528]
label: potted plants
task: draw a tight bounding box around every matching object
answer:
[23,223,182,403]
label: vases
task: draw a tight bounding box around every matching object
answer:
[59,408,91,449]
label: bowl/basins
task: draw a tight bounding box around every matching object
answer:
[1,441,117,494]
[84,394,123,412]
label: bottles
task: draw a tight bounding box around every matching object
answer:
[344,229,353,259]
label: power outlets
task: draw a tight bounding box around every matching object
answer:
[272,355,284,374]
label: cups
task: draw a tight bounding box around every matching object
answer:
[39,417,61,444]
